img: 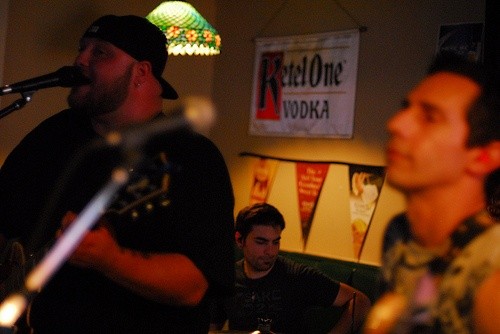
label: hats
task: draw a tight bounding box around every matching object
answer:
[81,15,179,101]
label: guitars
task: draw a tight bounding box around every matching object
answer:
[0,149,183,334]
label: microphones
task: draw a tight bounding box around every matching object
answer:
[0,66,84,96]
[97,95,216,152]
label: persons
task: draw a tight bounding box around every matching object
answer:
[364,49,500,334]
[0,14,235,334]
[206,203,371,334]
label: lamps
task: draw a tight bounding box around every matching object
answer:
[145,0,222,56]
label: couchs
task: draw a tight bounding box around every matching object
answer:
[229,241,382,334]
